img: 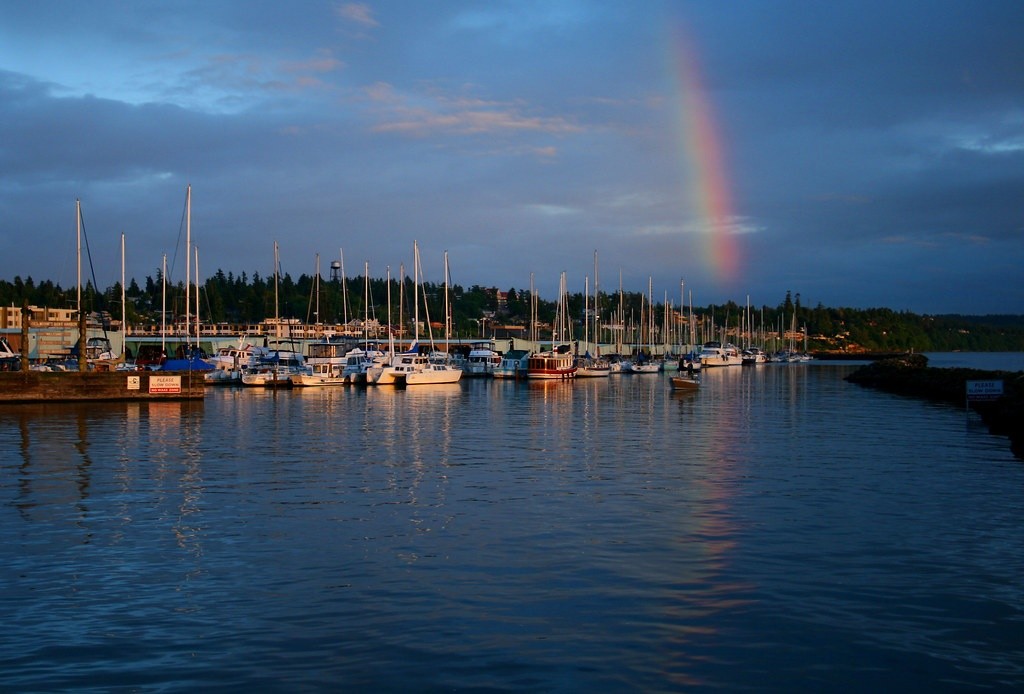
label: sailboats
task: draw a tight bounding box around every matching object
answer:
[4,185,813,386]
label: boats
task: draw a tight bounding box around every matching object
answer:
[669,362,704,392]
